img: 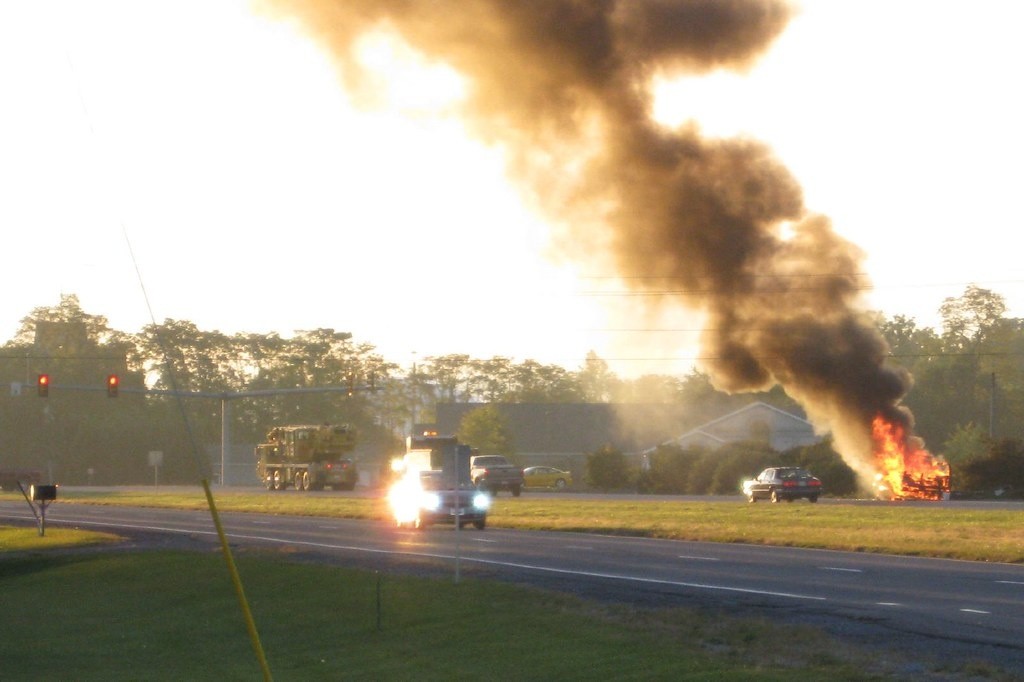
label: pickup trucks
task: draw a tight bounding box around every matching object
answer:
[471,454,525,496]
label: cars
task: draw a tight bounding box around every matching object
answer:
[744,467,825,504]
[393,469,487,531]
[523,466,574,491]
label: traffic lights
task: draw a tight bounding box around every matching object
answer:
[37,374,49,397]
[106,373,119,399]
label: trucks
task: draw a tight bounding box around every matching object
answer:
[404,432,471,483]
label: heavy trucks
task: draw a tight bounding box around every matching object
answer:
[254,424,359,493]
